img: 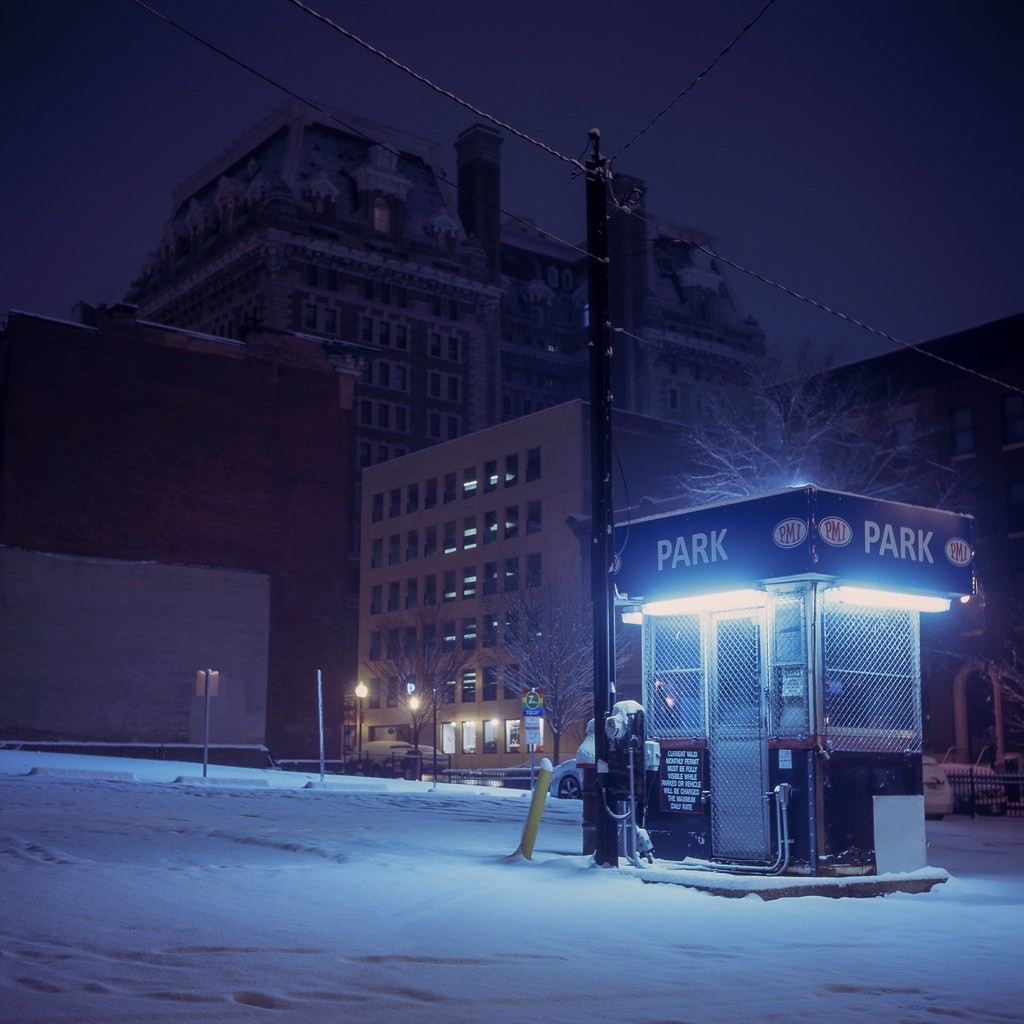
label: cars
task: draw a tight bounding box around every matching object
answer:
[341,741,447,777]
[922,758,1006,821]
[501,753,577,790]
[551,758,586,800]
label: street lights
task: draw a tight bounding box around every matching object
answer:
[355,680,368,772]
